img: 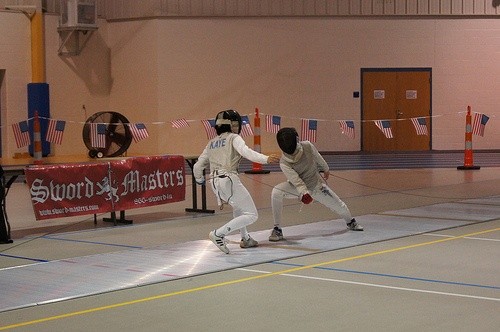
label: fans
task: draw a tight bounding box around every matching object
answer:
[83,111,133,159]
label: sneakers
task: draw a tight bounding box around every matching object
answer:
[269,229,283,241]
[239,234,258,248]
[209,229,230,254]
[347,218,364,231]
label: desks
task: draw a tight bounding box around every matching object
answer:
[0,155,215,244]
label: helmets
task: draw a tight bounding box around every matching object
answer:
[214,109,241,135]
[276,127,297,154]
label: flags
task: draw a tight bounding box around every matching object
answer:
[12,120,30,148]
[374,121,394,138]
[90,124,106,148]
[337,121,356,137]
[300,119,317,143]
[128,123,150,143]
[412,118,428,135]
[169,118,190,129]
[473,113,489,137]
[264,115,281,134]
[201,120,218,140]
[240,116,254,138]
[47,120,66,144]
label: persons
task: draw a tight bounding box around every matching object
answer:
[193,110,280,255]
[269,127,364,241]
